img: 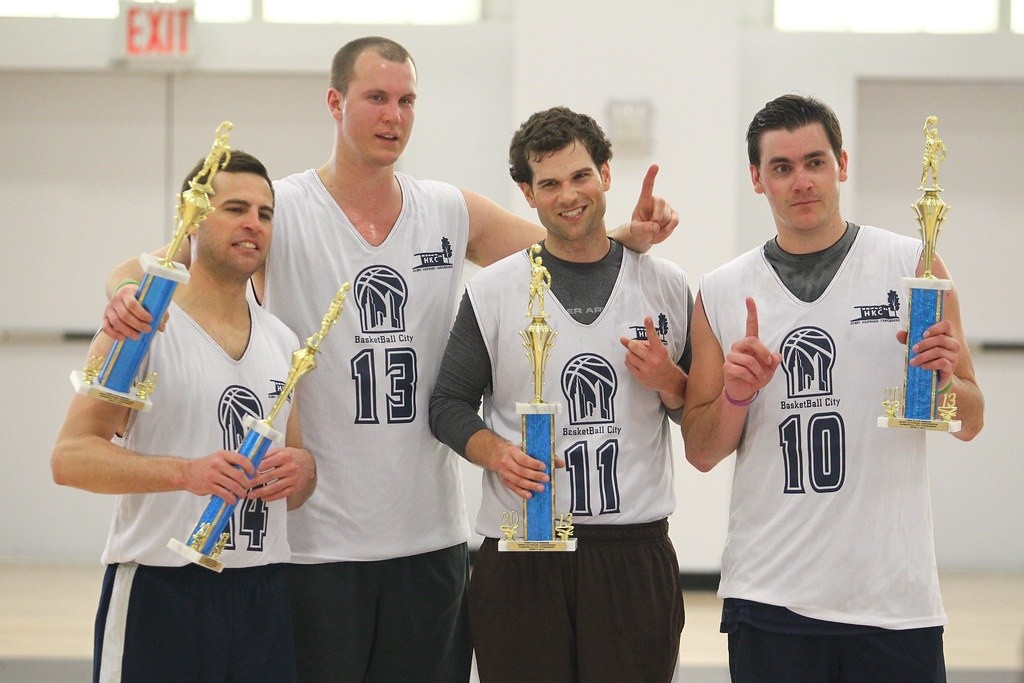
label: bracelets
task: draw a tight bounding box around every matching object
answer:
[725,388,759,406]
[112,279,141,293]
[938,378,953,396]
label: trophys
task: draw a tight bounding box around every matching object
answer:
[876,115,962,433]
[68,121,233,411]
[166,282,352,573]
[497,244,578,551]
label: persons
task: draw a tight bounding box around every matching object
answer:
[100,36,680,683]
[428,107,695,683]
[680,94,984,683]
[50,149,317,683]
